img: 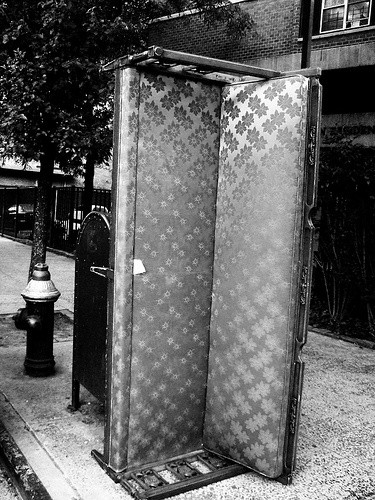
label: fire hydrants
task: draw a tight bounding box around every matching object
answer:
[10,262,61,378]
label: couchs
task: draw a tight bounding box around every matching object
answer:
[90,45,324,500]
